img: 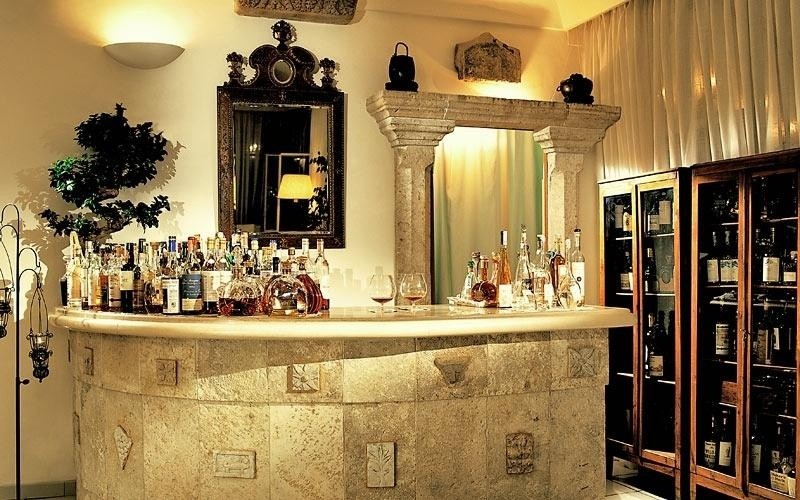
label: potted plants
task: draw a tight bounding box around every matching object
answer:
[36,102,172,309]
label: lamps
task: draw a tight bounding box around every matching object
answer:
[102,42,186,73]
[274,171,316,205]
[0,201,54,498]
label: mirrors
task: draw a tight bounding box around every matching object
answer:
[214,18,346,250]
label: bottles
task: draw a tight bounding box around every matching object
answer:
[66,232,331,315]
[607,196,673,296]
[701,185,796,497]
[462,230,586,313]
[642,310,668,380]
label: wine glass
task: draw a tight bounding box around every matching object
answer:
[364,274,428,313]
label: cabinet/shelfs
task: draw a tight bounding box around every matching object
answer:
[687,149,799,500]
[594,167,687,499]
